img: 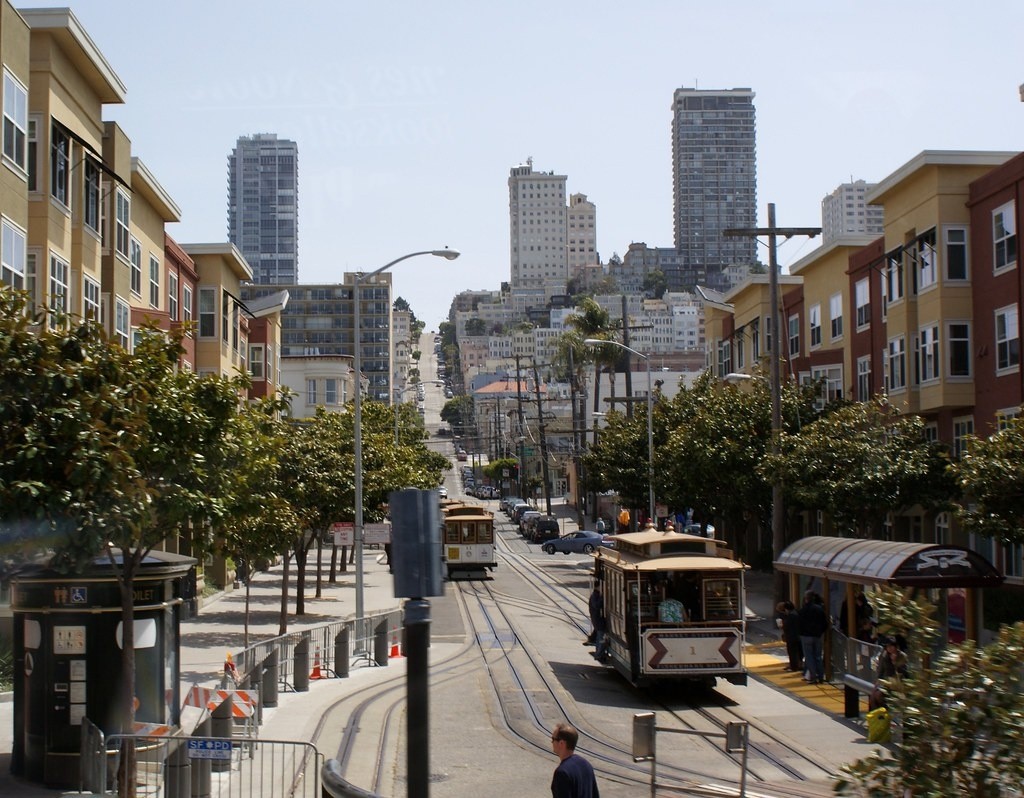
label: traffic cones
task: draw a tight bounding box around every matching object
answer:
[388,626,404,658]
[309,646,325,679]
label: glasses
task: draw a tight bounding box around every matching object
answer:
[552,737,565,743]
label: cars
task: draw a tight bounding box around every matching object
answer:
[540,530,613,556]
[439,486,449,500]
[452,426,466,461]
[460,464,498,498]
[416,334,453,410]
[499,497,543,535]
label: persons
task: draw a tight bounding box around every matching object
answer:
[549,723,600,798]
[863,636,910,729]
[595,517,605,535]
[779,583,880,684]
[588,579,736,660]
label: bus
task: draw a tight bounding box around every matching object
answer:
[588,519,748,689]
[439,498,498,574]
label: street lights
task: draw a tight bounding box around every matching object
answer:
[395,380,447,450]
[581,337,655,528]
[349,248,464,652]
[726,370,787,627]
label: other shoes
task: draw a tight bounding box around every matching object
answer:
[787,667,796,671]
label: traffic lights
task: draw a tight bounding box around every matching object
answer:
[509,464,518,479]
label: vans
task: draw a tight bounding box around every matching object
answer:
[527,515,560,543]
